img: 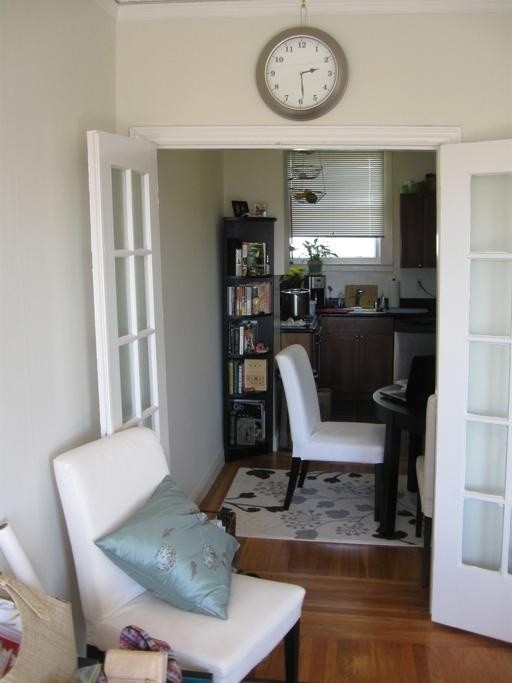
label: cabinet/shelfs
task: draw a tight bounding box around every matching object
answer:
[221,216,277,464]
[280,322,321,390]
[399,192,436,272]
[321,317,393,402]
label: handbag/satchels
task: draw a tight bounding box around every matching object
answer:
[0,572,78,682]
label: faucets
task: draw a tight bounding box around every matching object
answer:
[376,296,385,308]
[355,288,364,307]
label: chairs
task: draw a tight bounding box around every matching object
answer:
[391,330,436,382]
[273,343,388,521]
[415,394,436,590]
[52,427,307,683]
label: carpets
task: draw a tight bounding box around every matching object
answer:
[214,467,425,547]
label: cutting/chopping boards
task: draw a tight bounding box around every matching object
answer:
[344,284,378,308]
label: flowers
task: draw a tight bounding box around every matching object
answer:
[279,265,305,291]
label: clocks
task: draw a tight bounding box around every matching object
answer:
[255,26,349,121]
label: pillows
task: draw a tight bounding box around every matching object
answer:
[95,474,241,620]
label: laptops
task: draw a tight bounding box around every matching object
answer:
[379,353,434,407]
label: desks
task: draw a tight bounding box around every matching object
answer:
[371,383,435,537]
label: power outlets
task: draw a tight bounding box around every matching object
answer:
[416,279,425,290]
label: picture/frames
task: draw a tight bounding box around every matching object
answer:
[231,200,249,218]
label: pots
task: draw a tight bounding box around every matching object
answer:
[281,288,308,316]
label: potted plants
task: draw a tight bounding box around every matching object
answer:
[303,239,338,275]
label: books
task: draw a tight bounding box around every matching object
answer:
[226,241,271,445]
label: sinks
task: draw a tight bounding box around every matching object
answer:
[358,308,429,314]
